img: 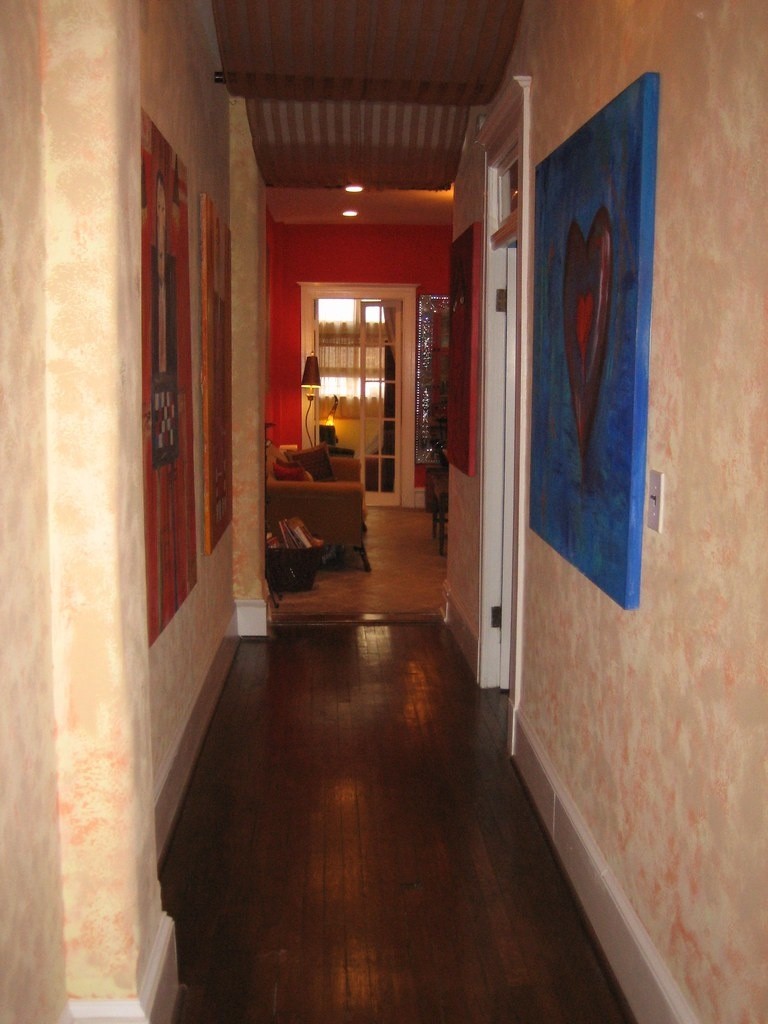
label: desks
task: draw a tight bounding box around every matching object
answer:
[425,467,449,555]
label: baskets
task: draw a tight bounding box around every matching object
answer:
[265,539,324,592]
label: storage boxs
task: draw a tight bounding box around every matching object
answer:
[266,532,324,592]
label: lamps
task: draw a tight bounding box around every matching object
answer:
[300,351,322,447]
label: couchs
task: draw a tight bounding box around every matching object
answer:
[265,440,372,572]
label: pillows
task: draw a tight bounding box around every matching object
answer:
[272,462,311,482]
[283,440,336,482]
[275,457,300,468]
